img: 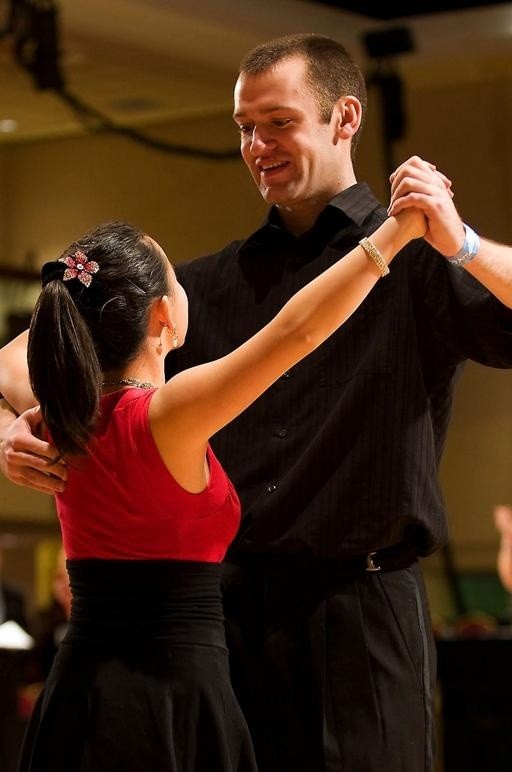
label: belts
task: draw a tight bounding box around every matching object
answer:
[224,544,425,579]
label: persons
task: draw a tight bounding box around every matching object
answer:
[0,30,512,771]
[0,503,510,770]
[0,158,457,772]
[0,30,512,772]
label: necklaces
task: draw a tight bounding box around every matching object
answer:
[94,374,156,393]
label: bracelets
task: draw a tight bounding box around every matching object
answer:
[445,223,481,268]
[358,236,390,278]
